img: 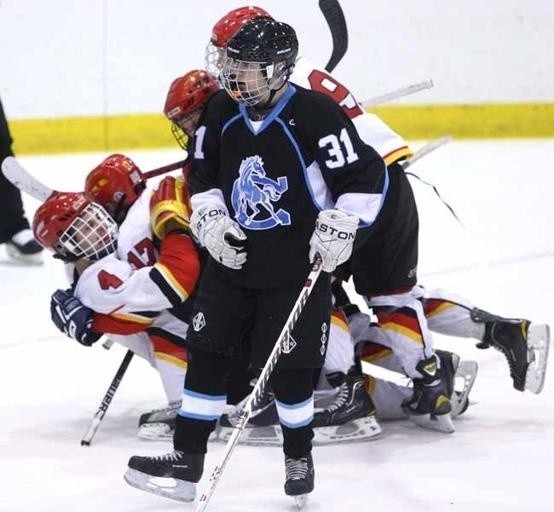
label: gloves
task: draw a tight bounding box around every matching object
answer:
[50,290,104,345]
[189,206,248,270]
[308,209,360,273]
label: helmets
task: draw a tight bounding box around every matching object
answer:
[164,7,299,151]
[35,193,119,261]
[82,155,147,214]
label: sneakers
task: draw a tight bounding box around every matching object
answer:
[126,451,204,483]
[305,343,383,446]
[217,379,284,446]
[431,347,478,418]
[400,355,456,433]
[137,398,183,441]
[283,452,314,495]
[12,230,43,255]
[469,309,551,395]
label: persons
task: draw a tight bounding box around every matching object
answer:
[0,6,532,508]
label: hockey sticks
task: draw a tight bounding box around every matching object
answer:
[319,0,348,72]
[1,80,434,204]
[402,136,451,172]
